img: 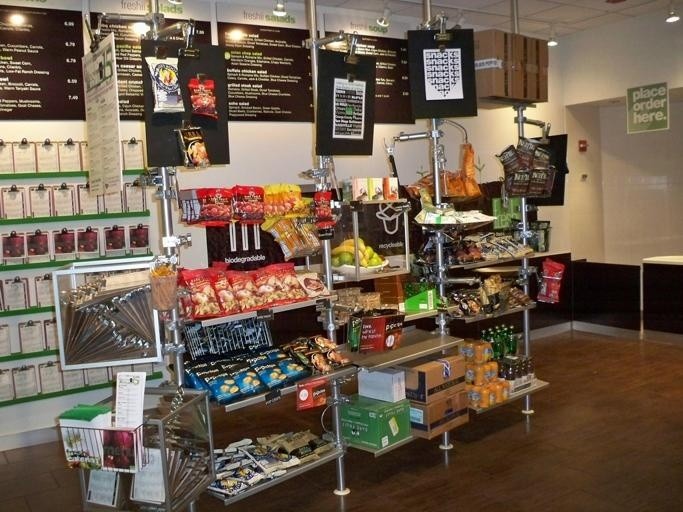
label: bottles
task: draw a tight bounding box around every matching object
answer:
[461,337,491,364]
[480,324,517,360]
[464,360,498,386]
[466,379,510,408]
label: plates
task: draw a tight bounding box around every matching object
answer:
[332,259,389,274]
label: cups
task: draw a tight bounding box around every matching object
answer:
[150,273,177,310]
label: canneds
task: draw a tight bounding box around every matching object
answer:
[499,356,534,395]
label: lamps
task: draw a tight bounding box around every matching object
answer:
[375,6,391,27]
[664,4,679,22]
[547,28,557,46]
[272,0,286,16]
[167,0,181,5]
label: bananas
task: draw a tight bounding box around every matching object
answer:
[339,238,365,253]
[331,245,363,254]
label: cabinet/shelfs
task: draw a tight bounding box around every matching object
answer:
[414,251,642,342]
[51,0,565,511]
[641,255,682,347]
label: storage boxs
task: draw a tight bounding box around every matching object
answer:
[473,28,548,103]
[396,353,465,405]
[357,368,405,404]
[338,393,410,450]
[379,294,404,310]
[408,389,469,440]
[373,272,411,283]
[373,281,404,297]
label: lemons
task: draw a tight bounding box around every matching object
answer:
[339,251,354,265]
[366,246,373,258]
[371,252,382,266]
[331,256,340,266]
